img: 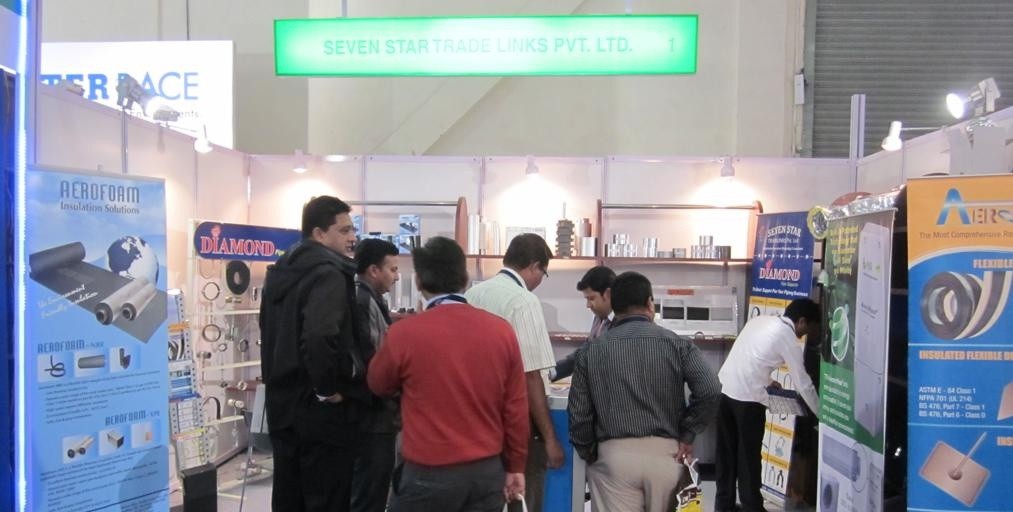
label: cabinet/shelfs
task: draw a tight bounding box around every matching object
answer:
[197,195,763,468]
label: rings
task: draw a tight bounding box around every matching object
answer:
[683,455,686,458]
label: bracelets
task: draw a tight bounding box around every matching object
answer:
[540,433,557,440]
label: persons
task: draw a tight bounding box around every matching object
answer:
[347,237,410,511]
[547,265,619,382]
[258,196,383,512]
[366,236,532,512]
[714,297,821,512]
[464,234,567,511]
[568,270,722,511]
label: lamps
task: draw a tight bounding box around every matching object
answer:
[524,155,539,176]
[115,73,166,176]
[880,120,943,155]
[944,76,1002,120]
[720,155,737,178]
[292,146,308,176]
